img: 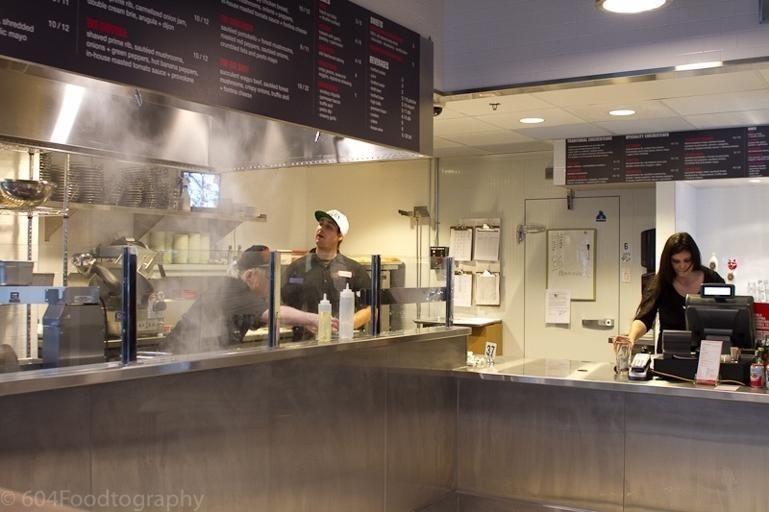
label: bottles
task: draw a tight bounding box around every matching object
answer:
[153,292,166,340]
[172,184,191,212]
[746,280,766,303]
[338,281,356,343]
[317,292,333,344]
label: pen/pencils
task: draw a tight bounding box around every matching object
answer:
[586,244,590,259]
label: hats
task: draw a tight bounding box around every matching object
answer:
[314,208,349,238]
[238,245,272,266]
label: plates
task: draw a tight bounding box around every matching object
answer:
[37,152,170,211]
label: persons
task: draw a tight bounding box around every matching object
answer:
[158,245,339,355]
[280,209,371,341]
[612,232,726,352]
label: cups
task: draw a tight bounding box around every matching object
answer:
[615,340,631,376]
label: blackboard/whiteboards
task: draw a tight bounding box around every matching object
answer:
[546,228,597,301]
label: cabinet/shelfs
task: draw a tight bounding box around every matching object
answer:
[22,150,178,216]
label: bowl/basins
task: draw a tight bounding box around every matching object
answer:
[0,177,58,209]
[149,230,210,264]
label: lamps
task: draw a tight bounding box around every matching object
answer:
[594,0,674,16]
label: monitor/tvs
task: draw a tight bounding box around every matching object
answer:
[685,294,755,354]
[180,170,221,212]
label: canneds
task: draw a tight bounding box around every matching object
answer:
[765,364,769,388]
[750,363,765,387]
